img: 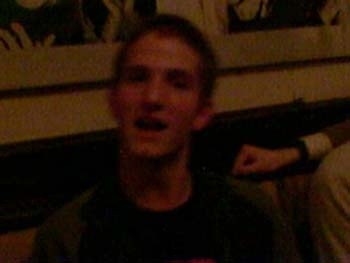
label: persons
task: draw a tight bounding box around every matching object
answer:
[232,111,349,262]
[30,14,291,263]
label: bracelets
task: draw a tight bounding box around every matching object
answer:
[291,137,310,160]
